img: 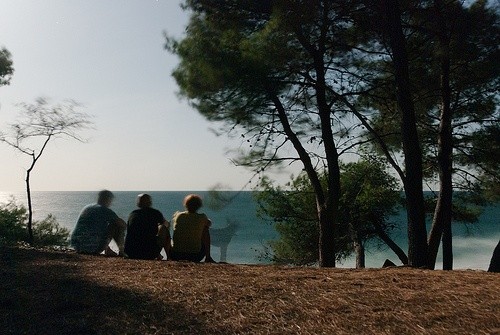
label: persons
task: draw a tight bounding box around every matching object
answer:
[124,194,172,260]
[169,194,216,263]
[70,189,128,257]
